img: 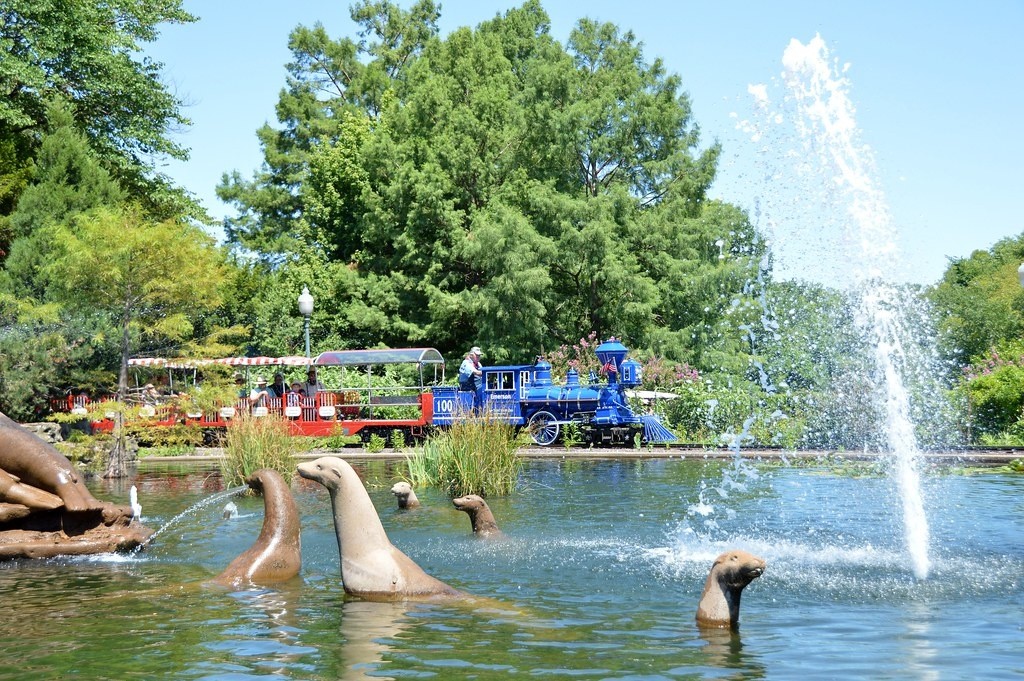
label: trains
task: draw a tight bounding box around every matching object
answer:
[39,342,679,446]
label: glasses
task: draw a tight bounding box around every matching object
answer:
[235,379,241,382]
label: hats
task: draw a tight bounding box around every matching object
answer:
[470,347,483,355]
[290,379,302,387]
[253,375,267,384]
[305,366,318,375]
[235,374,243,380]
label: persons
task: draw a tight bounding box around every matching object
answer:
[141,383,164,405]
[232,366,330,413]
[458,346,483,417]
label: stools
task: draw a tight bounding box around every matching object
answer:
[459,391,476,418]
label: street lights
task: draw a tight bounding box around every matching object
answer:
[296,284,315,358]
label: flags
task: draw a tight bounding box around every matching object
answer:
[598,356,617,376]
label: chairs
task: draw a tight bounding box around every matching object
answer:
[316,392,337,421]
[218,399,237,423]
[282,393,303,422]
[343,391,360,419]
[252,394,269,420]
[137,403,155,421]
[185,401,204,423]
[71,408,87,415]
[101,407,116,423]
